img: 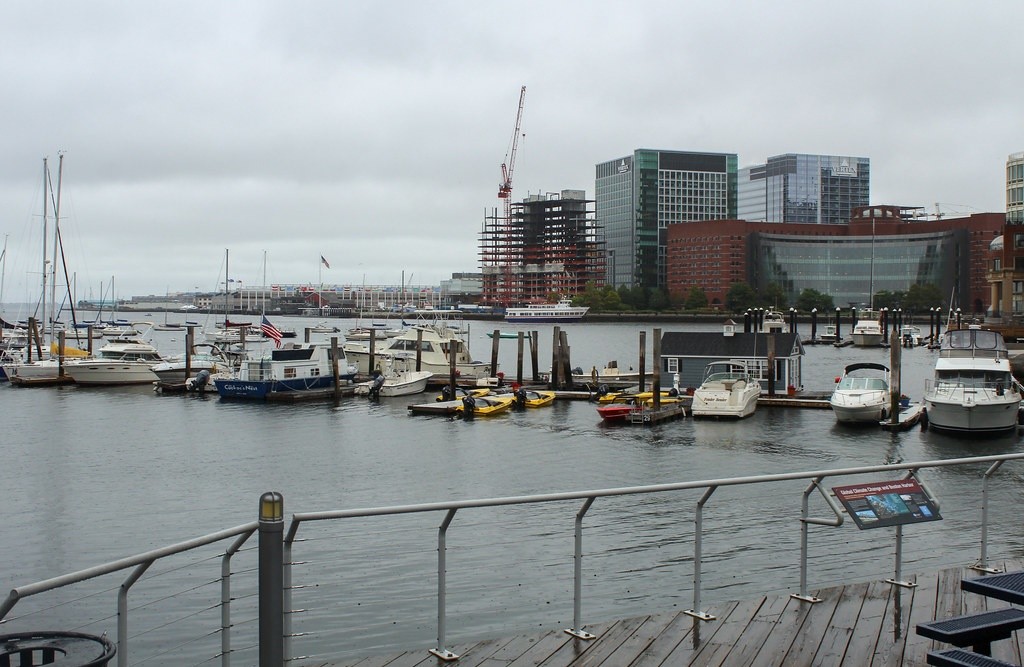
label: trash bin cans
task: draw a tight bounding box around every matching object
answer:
[686,386,696,396]
[512,382,521,391]
[787,386,795,398]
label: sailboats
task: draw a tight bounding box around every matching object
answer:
[1,157,137,387]
[201,248,298,342]
[153,288,202,331]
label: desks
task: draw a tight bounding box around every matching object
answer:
[960,568,1024,606]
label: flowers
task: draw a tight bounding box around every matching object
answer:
[899,394,909,399]
[686,387,697,392]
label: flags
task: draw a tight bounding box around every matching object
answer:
[261,314,283,349]
[321,255,329,268]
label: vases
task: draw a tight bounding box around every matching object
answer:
[688,390,694,396]
[899,398,911,407]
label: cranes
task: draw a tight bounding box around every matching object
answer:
[498,85,527,307]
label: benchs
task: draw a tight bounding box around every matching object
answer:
[915,606,1024,657]
[927,647,1021,667]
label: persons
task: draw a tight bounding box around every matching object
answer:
[376,361,381,369]
[592,365,598,383]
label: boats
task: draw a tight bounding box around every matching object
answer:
[344,305,500,378]
[821,325,843,340]
[596,403,640,423]
[849,320,883,347]
[354,354,433,397]
[830,362,891,427]
[487,332,532,339]
[504,300,590,323]
[923,329,1023,438]
[690,361,762,420]
[308,322,335,333]
[455,397,511,417]
[212,342,358,399]
[511,391,555,408]
[900,326,923,347]
[598,392,685,407]
[60,339,171,385]
[148,343,257,385]
